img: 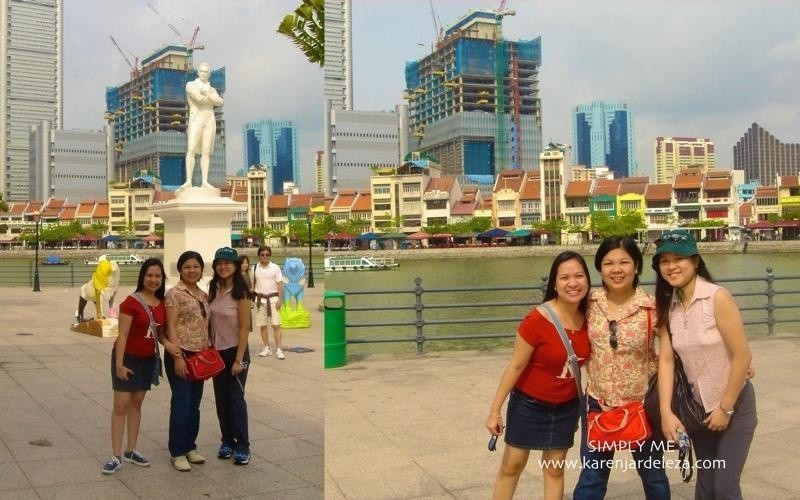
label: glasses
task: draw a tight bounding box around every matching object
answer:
[259,253,271,256]
[608,318,618,350]
[489,420,505,453]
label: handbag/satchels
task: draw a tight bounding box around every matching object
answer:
[584,399,652,451]
[152,353,164,386]
[184,348,226,380]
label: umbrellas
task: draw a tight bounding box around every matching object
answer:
[231,234,242,242]
[72,233,163,248]
[328,225,533,246]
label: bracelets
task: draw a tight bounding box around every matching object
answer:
[490,412,503,420]
[235,360,244,365]
[718,402,735,416]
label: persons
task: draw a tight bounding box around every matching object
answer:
[177,62,225,189]
[248,245,287,360]
[572,233,757,499]
[650,226,757,499]
[238,254,253,334]
[484,251,594,499]
[163,249,212,473]
[207,246,253,467]
[99,257,183,477]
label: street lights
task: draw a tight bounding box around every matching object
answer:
[306,210,316,289]
[29,213,43,292]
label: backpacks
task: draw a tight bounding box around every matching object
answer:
[642,321,711,454]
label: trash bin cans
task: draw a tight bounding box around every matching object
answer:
[324,290,347,369]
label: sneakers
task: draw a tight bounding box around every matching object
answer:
[102,456,122,474]
[217,440,251,465]
[258,346,273,356]
[168,447,205,471]
[276,347,285,358]
[124,450,151,466]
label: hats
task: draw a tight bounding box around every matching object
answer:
[213,247,240,266]
[652,228,699,273]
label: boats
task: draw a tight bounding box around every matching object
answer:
[83,253,149,265]
[41,255,72,267]
[324,254,400,271]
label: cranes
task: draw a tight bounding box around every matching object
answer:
[427,0,445,49]
[498,0,509,11]
